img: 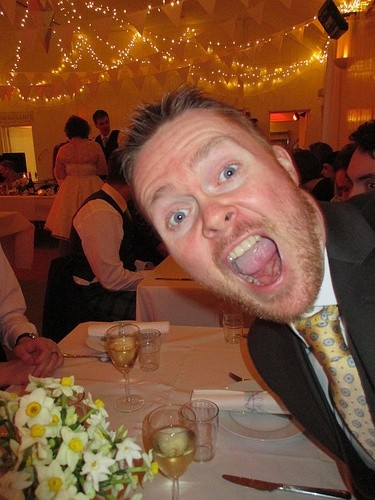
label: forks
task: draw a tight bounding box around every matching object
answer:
[229,372,251,382]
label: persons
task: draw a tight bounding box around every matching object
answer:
[1,246,63,391]
[124,85,375,500]
[1,106,375,335]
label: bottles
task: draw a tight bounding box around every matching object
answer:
[11,171,35,195]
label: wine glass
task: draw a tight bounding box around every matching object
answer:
[147,404,199,500]
[107,324,145,412]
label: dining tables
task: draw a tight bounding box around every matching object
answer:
[135,254,257,328]
[0,194,59,269]
[0,320,354,500]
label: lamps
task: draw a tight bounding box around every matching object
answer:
[292,111,306,121]
[316,0,357,41]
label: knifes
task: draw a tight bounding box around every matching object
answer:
[222,474,351,500]
[154,277,192,282]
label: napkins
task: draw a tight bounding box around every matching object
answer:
[88,321,170,340]
[191,389,289,415]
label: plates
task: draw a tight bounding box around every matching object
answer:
[217,378,306,440]
[86,336,108,353]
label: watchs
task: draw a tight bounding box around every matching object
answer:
[15,332,38,346]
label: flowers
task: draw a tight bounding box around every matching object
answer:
[1,373,160,500]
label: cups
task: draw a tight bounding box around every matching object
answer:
[222,306,244,344]
[183,399,219,464]
[135,329,161,372]
[1,183,9,195]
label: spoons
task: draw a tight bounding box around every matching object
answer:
[63,353,111,363]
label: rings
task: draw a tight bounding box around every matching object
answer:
[51,351,59,356]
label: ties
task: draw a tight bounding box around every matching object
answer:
[294,305,375,464]
[102,137,107,147]
